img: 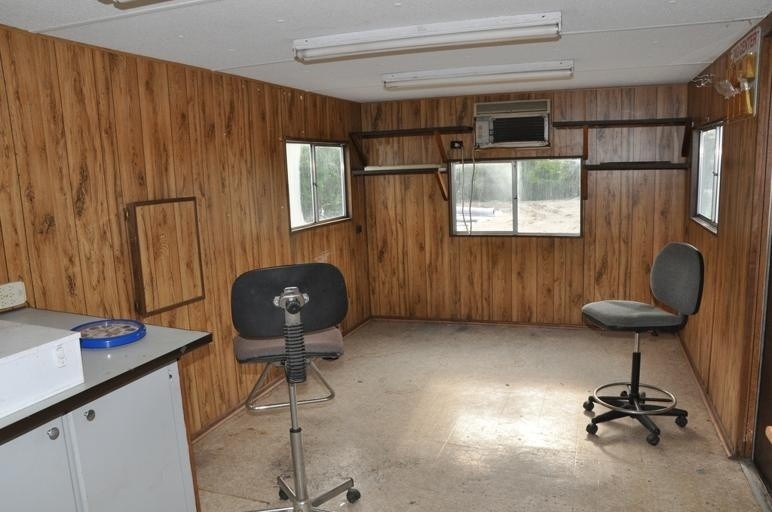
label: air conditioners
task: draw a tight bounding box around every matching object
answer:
[470,99,561,153]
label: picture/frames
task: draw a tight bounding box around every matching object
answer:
[125,195,206,320]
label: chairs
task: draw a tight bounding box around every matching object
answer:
[581,236,708,447]
[225,261,361,512]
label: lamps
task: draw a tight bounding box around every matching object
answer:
[379,59,575,91]
[286,7,567,66]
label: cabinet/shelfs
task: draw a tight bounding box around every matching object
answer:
[552,115,695,200]
[349,121,473,204]
[0,361,196,512]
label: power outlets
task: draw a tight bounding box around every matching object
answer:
[450,140,464,150]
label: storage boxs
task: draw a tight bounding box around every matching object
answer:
[0,320,84,419]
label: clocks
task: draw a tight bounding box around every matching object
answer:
[69,318,146,349]
